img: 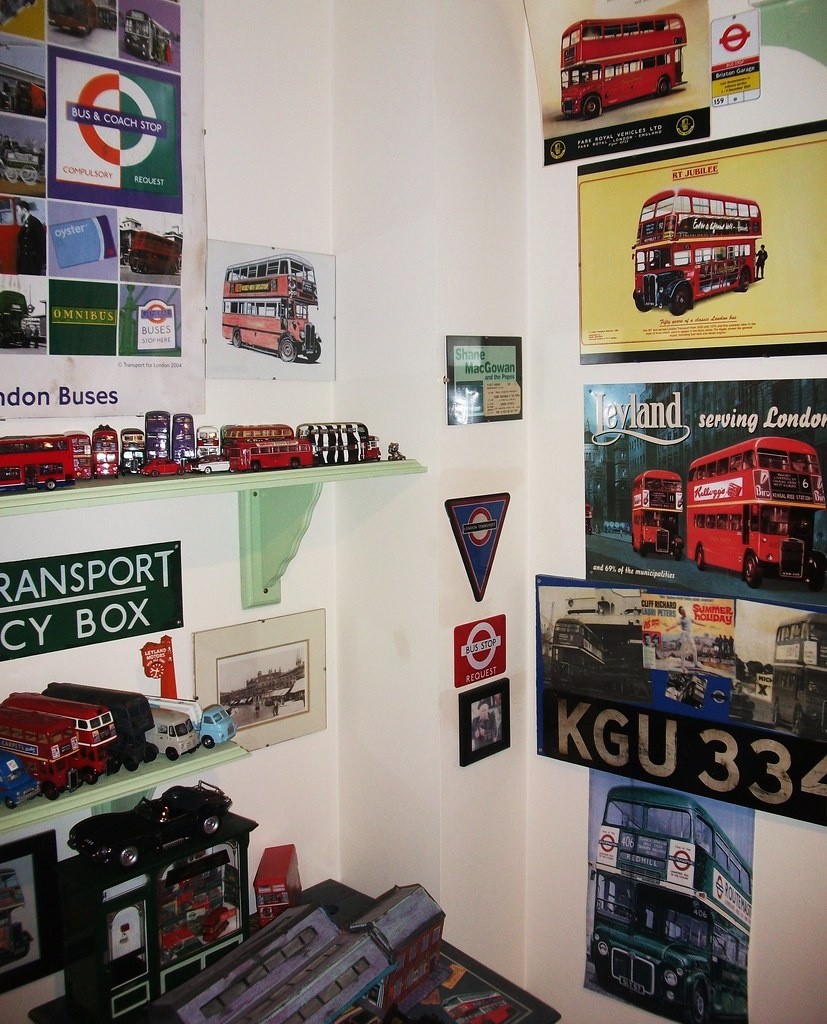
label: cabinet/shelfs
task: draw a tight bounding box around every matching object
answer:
[0,457,434,828]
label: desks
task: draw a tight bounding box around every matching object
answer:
[25,876,561,1024]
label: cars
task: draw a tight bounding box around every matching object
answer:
[191,455,235,475]
[139,457,186,478]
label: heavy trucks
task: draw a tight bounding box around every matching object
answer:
[297,422,381,465]
[141,694,237,751]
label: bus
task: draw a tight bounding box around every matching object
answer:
[551,618,606,690]
[0,434,75,492]
[254,844,303,928]
[119,427,147,476]
[633,190,765,316]
[1,704,85,802]
[771,612,827,742]
[197,426,219,459]
[119,8,171,65]
[62,430,93,480]
[590,783,752,1023]
[222,254,323,362]
[1,291,32,350]
[15,80,46,119]
[48,0,99,37]
[686,436,826,593]
[221,423,294,466]
[2,692,119,785]
[228,435,312,472]
[143,410,171,465]
[89,423,121,480]
[42,682,158,772]
[632,470,684,561]
[441,992,515,1024]
[172,414,196,472]
[560,13,689,119]
[95,5,117,31]
[128,232,178,275]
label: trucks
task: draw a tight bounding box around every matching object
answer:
[0,751,43,809]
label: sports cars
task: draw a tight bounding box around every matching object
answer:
[67,779,233,869]
